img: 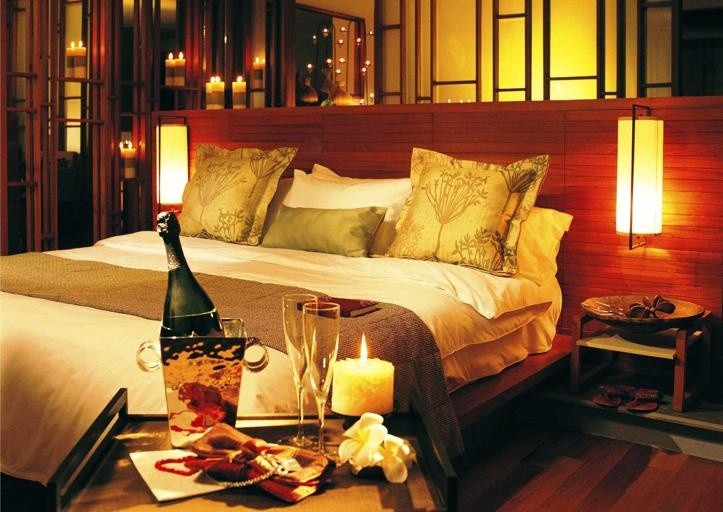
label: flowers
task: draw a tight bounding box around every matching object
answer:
[337,412,418,484]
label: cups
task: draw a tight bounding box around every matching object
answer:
[137,315,268,449]
[220,317,245,337]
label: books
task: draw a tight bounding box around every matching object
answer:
[297,296,381,318]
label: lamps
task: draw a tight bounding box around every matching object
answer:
[300,17,373,105]
[155,115,190,213]
[615,103,664,251]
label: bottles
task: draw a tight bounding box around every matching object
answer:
[156,211,225,338]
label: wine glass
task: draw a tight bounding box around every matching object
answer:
[302,303,346,467]
[277,293,317,448]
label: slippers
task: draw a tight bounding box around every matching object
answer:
[624,387,659,411]
[593,384,635,407]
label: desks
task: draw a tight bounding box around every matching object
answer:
[47,389,448,512]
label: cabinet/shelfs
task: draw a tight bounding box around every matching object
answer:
[570,296,713,413]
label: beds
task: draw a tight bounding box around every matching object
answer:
[0,231,573,497]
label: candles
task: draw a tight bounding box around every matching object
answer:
[74,40,87,79]
[165,53,174,86]
[232,75,247,110]
[65,40,73,78]
[253,54,263,88]
[124,141,136,179]
[174,53,186,87]
[212,75,225,110]
[206,75,213,110]
[332,332,394,417]
